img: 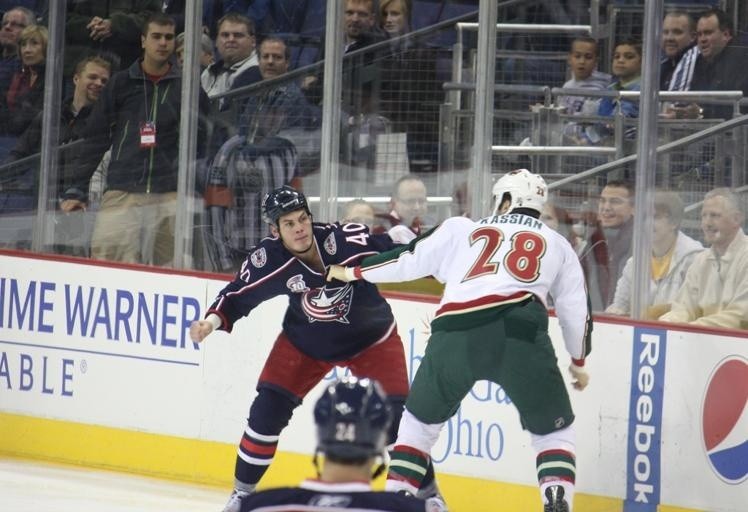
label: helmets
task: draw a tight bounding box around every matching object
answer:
[260,185,309,228]
[314,377,392,460]
[490,168,548,217]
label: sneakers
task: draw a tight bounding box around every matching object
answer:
[539,479,574,512]
[221,485,256,512]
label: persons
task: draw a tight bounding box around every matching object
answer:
[325,168,593,512]
[217,375,446,512]
[1,1,436,273]
[497,1,748,332]
[188,185,445,512]
